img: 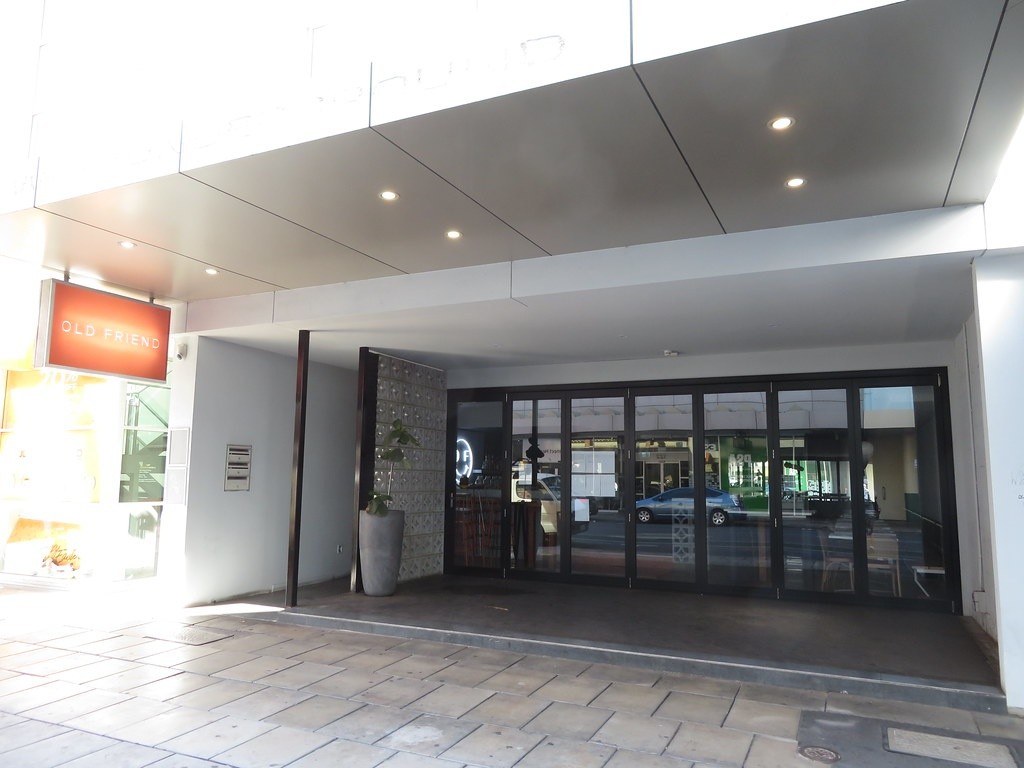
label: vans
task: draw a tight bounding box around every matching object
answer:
[472,469,588,546]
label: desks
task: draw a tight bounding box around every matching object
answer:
[829,533,899,565]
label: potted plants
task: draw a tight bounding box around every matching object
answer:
[359,417,423,597]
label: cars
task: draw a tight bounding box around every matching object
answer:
[636,486,747,525]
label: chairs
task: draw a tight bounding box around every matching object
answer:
[816,530,853,592]
[864,536,902,597]
